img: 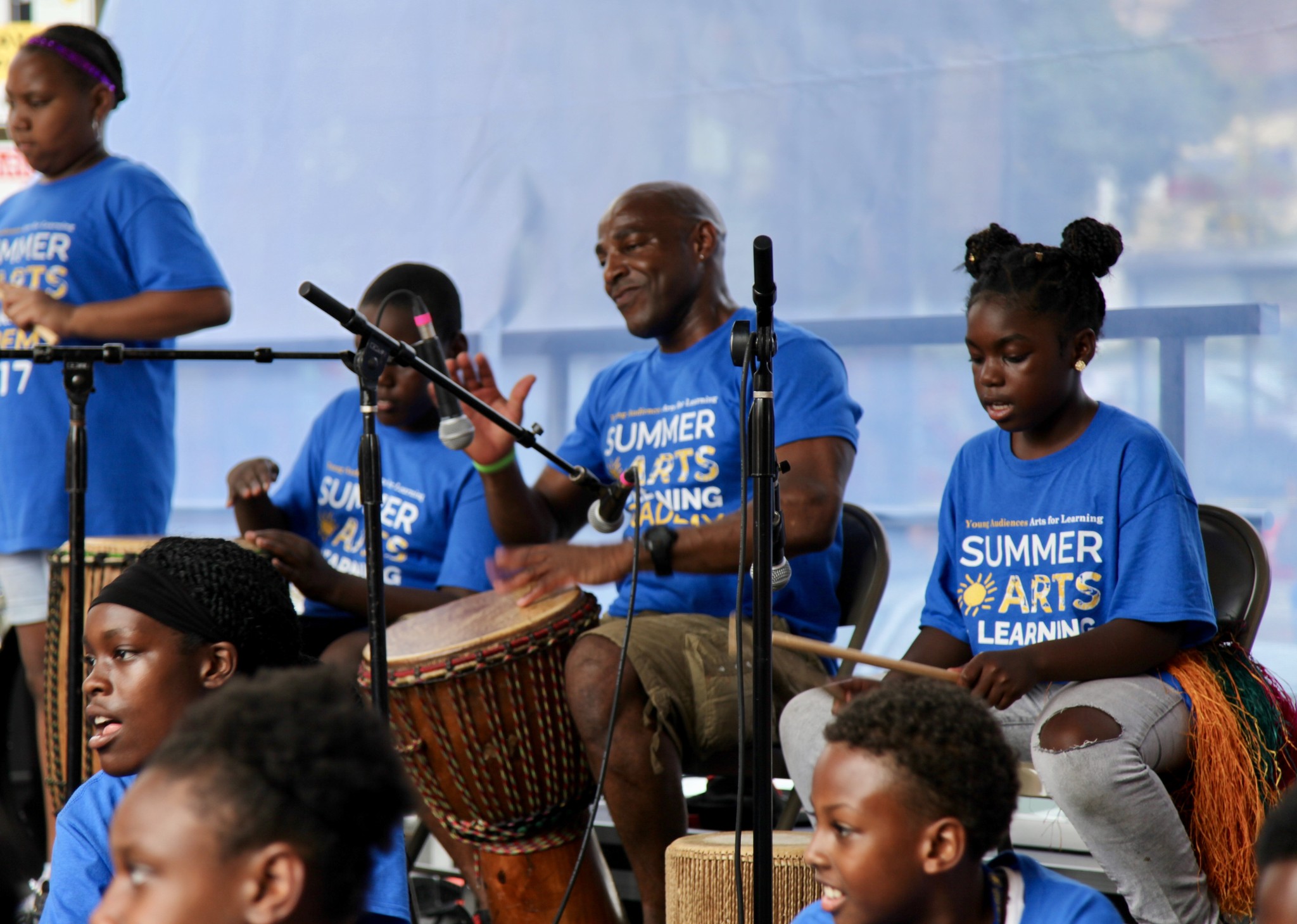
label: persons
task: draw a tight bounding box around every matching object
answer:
[776,217,1234,924]
[36,260,505,924]
[1253,788,1296,923]
[426,181,863,923]
[0,24,231,924]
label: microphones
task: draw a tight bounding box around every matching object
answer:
[749,471,793,591]
[414,296,475,451]
[587,469,636,534]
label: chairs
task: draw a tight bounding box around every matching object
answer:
[774,502,891,831]
[1197,504,1271,660]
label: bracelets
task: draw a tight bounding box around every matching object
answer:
[468,450,519,477]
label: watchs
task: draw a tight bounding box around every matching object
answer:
[642,523,679,578]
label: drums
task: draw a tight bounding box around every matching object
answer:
[663,827,830,924]
[41,532,270,818]
[359,580,628,923]
